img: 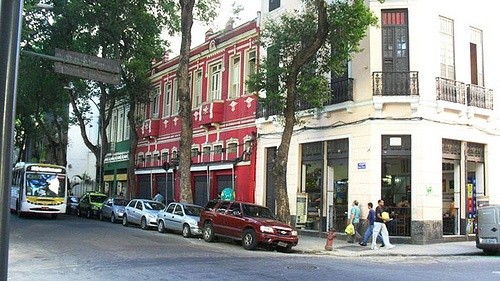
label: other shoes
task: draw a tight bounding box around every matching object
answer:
[380,244,385,247]
[347,240,355,243]
[370,246,379,250]
[387,245,395,249]
[360,244,366,246]
[359,241,362,244]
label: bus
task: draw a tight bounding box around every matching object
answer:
[10,161,68,218]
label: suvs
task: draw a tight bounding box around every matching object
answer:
[197,199,299,253]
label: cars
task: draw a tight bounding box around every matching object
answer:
[66,196,81,215]
[99,196,133,223]
[76,191,110,218]
[121,199,166,230]
[156,202,204,238]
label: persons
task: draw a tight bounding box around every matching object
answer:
[386,196,409,206]
[450,197,454,221]
[359,203,385,247]
[120,193,125,198]
[217,190,221,199]
[371,200,395,250]
[347,200,363,242]
[153,192,164,202]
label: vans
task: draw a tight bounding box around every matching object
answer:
[476,203,500,254]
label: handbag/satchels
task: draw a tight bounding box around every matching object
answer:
[382,211,390,222]
[344,224,355,235]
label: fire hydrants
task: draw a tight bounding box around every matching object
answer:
[324,226,337,251]
